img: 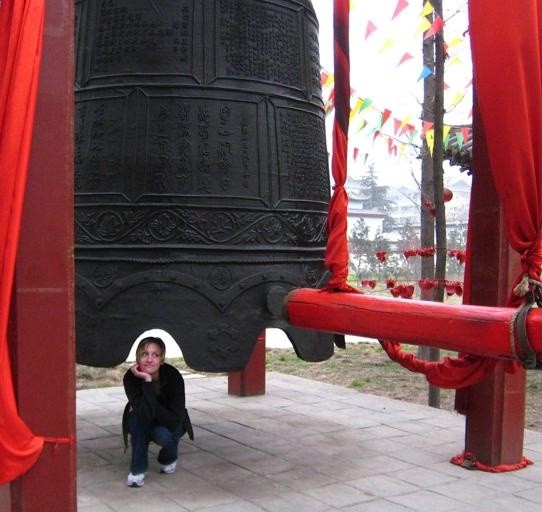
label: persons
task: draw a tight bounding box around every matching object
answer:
[119,334,196,487]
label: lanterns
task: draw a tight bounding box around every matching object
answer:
[444,188,453,201]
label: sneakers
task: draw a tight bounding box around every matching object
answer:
[160,460,177,474]
[128,472,146,487]
